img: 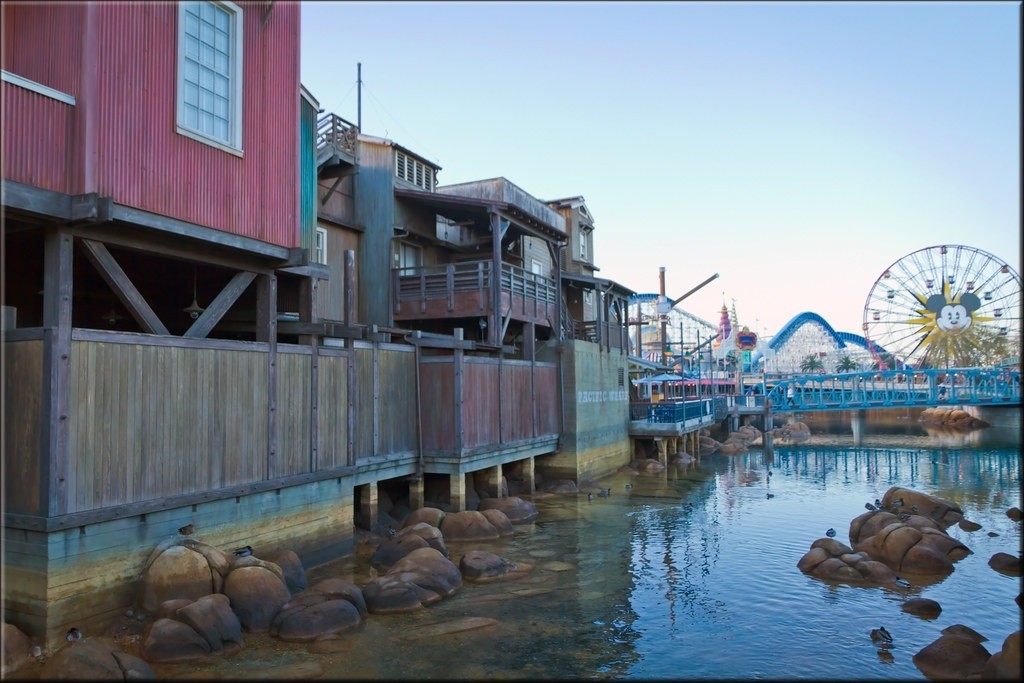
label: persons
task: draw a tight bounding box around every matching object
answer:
[787,384,795,406]
[937,381,947,400]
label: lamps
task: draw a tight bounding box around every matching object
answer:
[182,269,204,319]
[101,293,125,325]
